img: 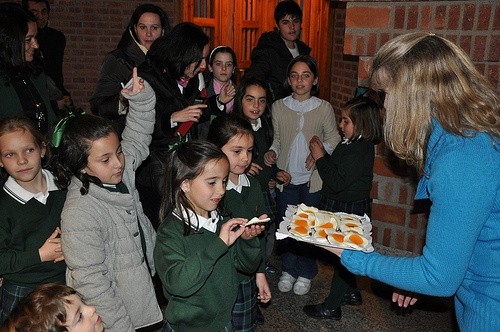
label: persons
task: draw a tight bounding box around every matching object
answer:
[0,0,382,332]
[313,32,500,332]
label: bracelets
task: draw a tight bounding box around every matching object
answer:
[216,96,225,105]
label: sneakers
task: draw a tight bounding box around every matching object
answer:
[293,276,311,295]
[278,271,296,292]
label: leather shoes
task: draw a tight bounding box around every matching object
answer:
[325,291,362,306]
[303,302,342,321]
